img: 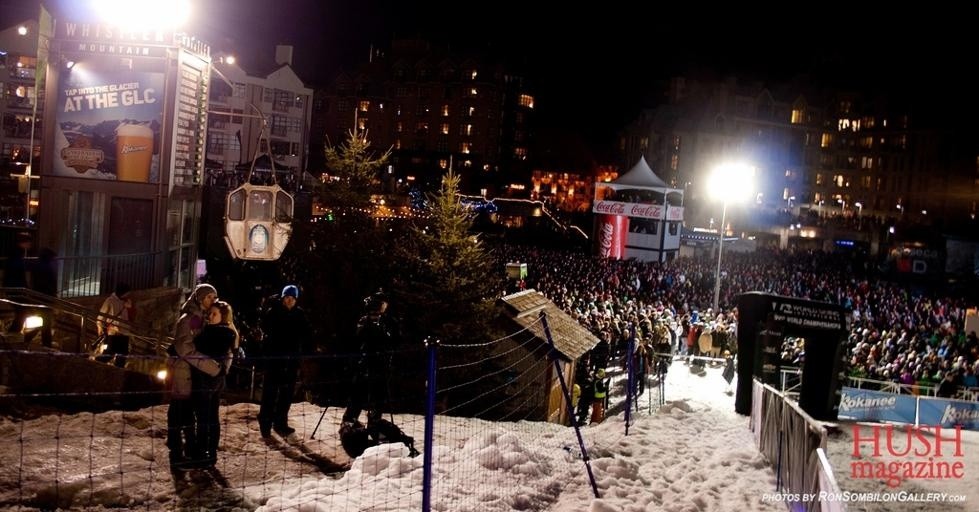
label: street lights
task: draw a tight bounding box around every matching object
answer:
[699,168,759,316]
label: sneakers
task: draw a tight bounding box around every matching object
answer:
[260,426,270,438]
[273,426,294,434]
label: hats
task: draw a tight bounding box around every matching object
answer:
[282,285,298,298]
[115,281,130,296]
[196,284,216,301]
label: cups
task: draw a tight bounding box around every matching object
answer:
[115,124,154,183]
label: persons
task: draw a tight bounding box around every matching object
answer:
[338,293,393,423]
[25,245,59,348]
[165,301,241,467]
[4,231,35,333]
[90,283,133,368]
[162,284,235,454]
[254,284,299,438]
[481,190,979,425]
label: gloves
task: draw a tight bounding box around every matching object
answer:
[238,346,244,358]
